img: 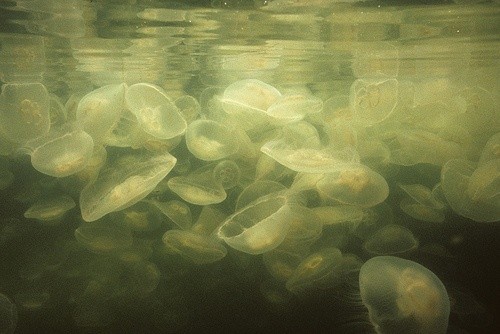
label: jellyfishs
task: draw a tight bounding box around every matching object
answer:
[0,1,499,334]
